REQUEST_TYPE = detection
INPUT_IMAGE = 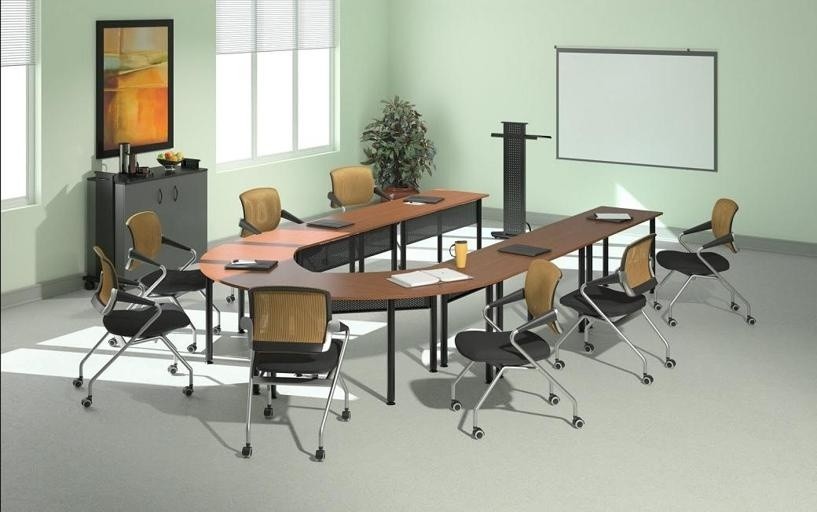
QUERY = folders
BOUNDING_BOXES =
[224,257,278,271]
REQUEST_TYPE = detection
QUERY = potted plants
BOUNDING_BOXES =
[362,94,439,203]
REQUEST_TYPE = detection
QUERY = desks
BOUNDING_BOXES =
[199,189,662,405]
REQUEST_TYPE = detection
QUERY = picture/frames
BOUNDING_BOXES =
[95,18,173,159]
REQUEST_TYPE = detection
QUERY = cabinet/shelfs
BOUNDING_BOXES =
[85,160,207,291]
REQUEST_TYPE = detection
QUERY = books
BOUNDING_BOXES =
[224,259,279,272]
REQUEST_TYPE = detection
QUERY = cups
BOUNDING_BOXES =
[449,241,468,269]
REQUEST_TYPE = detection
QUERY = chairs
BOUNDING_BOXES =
[125,211,222,354]
[450,260,584,438]
[560,235,676,385]
[226,187,305,303]
[242,286,353,462]
[74,245,194,408]
[653,199,756,328]
[326,167,391,209]
